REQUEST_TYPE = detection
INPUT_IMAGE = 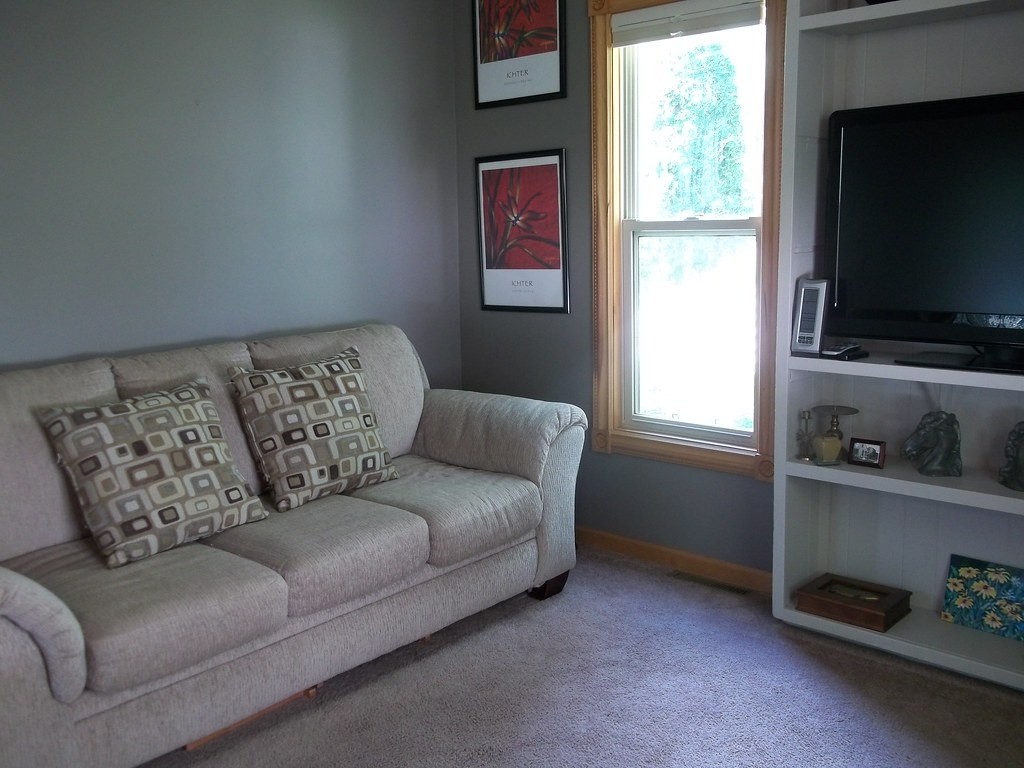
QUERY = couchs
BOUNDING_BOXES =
[0,323,588,768]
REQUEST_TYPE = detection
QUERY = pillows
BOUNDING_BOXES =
[35,375,269,569]
[229,345,399,512]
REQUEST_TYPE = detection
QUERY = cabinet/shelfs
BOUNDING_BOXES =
[771,0,1024,692]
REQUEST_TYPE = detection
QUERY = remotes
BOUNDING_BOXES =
[822,342,862,356]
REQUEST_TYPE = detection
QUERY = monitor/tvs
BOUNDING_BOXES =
[825,92,1024,374]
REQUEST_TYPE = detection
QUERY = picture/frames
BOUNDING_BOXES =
[847,438,886,469]
[475,148,570,314]
[471,0,566,110]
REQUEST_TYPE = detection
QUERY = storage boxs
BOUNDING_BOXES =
[795,572,912,633]
[941,554,1024,642]
